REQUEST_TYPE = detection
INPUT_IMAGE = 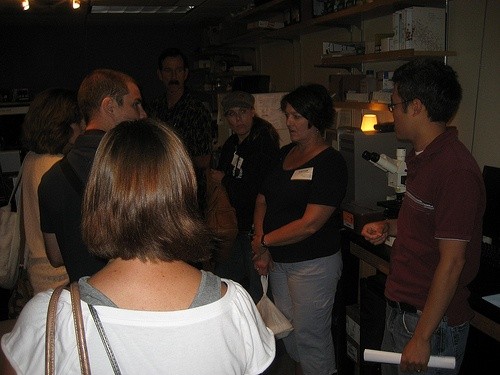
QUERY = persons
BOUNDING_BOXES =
[37,69,147,284]
[146,49,211,209]
[0,116,277,375]
[212,91,279,206]
[13,89,84,296]
[359,59,486,375]
[250,83,348,375]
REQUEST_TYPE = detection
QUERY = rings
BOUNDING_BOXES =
[417,368,421,374]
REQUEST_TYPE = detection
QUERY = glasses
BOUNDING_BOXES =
[387,99,412,112]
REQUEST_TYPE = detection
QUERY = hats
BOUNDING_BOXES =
[221,92,255,111]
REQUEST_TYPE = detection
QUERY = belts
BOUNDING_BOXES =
[385,298,418,315]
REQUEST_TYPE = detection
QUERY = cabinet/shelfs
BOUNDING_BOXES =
[201,0,464,73]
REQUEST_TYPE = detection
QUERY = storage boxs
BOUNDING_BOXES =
[191,51,254,73]
[329,75,401,135]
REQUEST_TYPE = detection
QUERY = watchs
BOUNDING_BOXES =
[260,233,271,250]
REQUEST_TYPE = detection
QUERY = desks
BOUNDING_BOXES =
[340,228,500,375]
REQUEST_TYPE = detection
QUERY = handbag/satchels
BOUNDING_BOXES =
[0,150,35,318]
